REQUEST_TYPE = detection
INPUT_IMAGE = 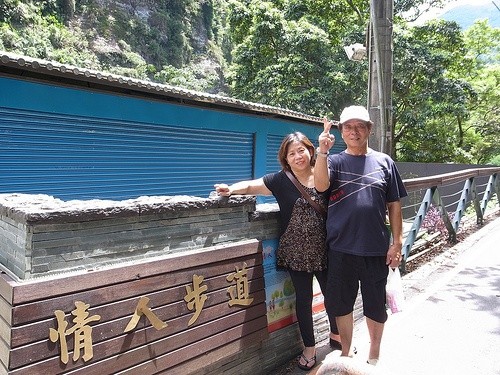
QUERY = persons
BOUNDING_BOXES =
[214,132,358,371]
[314,105,408,368]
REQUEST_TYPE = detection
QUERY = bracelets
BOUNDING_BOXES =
[317,147,329,157]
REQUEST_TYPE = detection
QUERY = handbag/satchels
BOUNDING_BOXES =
[385,264,403,314]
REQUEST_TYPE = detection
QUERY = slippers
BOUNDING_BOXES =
[297,351,316,371]
[330,338,357,354]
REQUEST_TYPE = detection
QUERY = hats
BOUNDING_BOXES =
[339,104,374,125]
